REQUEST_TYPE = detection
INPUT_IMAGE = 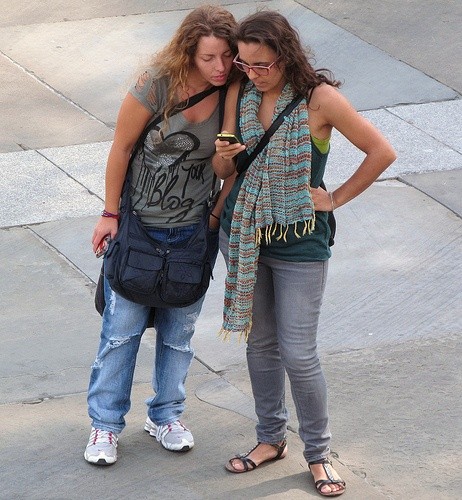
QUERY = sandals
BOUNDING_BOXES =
[225,439,288,473]
[308,459,346,496]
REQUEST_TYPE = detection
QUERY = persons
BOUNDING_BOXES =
[81,5,243,466]
[210,10,398,499]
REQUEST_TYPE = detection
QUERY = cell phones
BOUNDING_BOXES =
[217,134,241,145]
[95,234,111,258]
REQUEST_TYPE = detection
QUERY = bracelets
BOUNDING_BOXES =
[102,210,119,219]
[329,191,335,211]
[210,213,220,221]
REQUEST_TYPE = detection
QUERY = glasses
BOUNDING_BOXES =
[233,53,280,75]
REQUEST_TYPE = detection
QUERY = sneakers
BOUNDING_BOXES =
[143,417,195,452]
[84,427,118,465]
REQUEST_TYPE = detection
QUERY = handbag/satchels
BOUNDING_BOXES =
[104,209,213,308]
[95,261,157,329]
